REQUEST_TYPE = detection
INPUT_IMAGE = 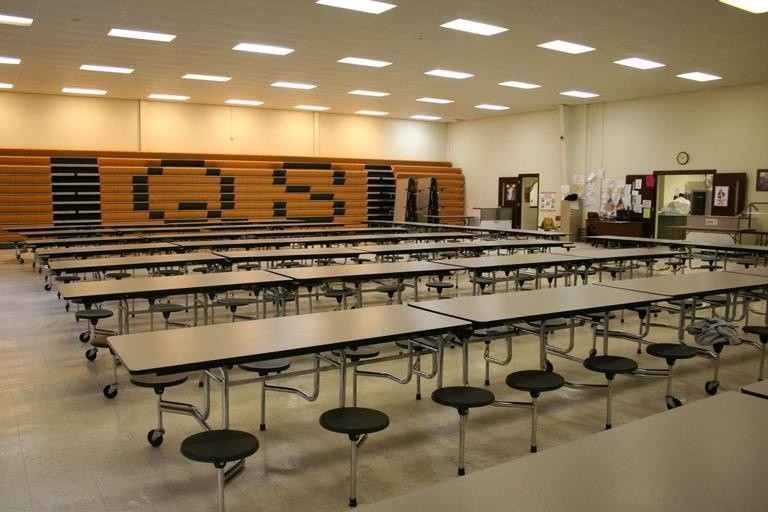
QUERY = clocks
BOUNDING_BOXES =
[677,152,689,165]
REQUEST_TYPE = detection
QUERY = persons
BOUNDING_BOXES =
[665,196,677,212]
[672,193,691,216]
[717,188,727,205]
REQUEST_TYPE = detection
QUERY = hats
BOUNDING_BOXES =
[674,186,684,197]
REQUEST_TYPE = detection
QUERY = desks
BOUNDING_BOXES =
[334,389,766,512]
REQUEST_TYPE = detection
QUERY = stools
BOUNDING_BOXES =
[9,211,766,450]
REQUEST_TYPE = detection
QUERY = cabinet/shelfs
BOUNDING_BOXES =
[559,200,583,244]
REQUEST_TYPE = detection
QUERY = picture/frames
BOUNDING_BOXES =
[756,168,768,191]
